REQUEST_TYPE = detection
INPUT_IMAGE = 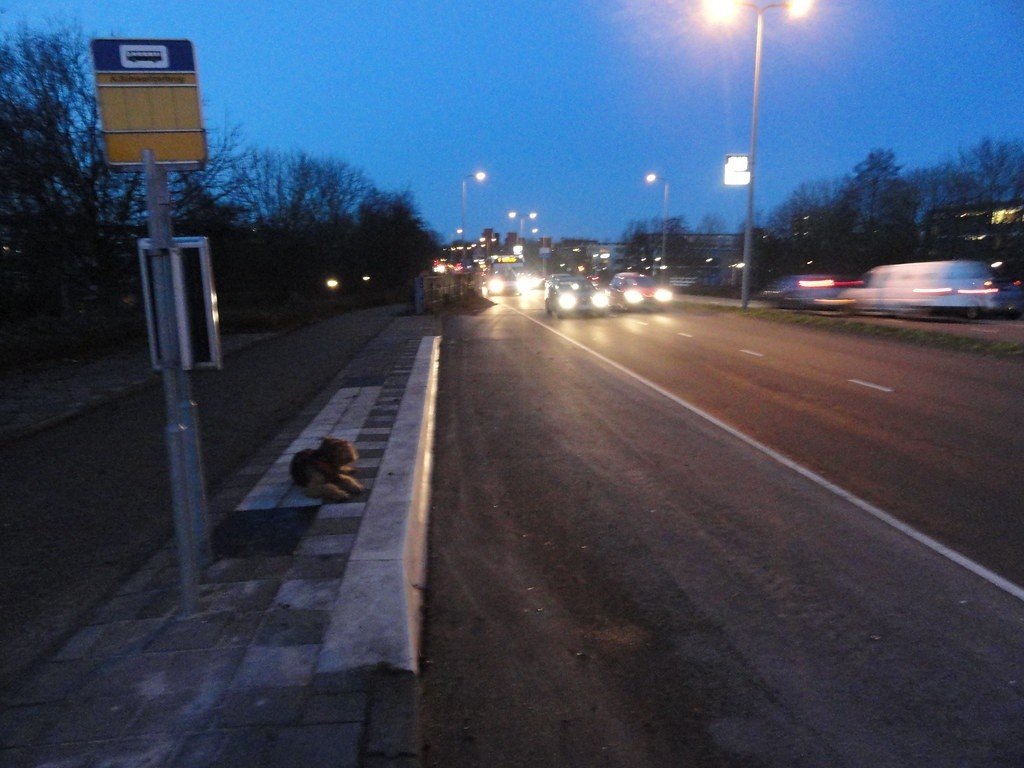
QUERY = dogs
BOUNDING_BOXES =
[288,435,365,501]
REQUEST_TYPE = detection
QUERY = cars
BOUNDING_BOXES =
[482,261,614,319]
[781,258,1024,320]
[614,271,677,316]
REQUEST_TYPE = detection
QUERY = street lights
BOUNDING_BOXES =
[695,0,818,310]
[646,173,669,263]
[509,210,538,256]
[459,168,486,261]
